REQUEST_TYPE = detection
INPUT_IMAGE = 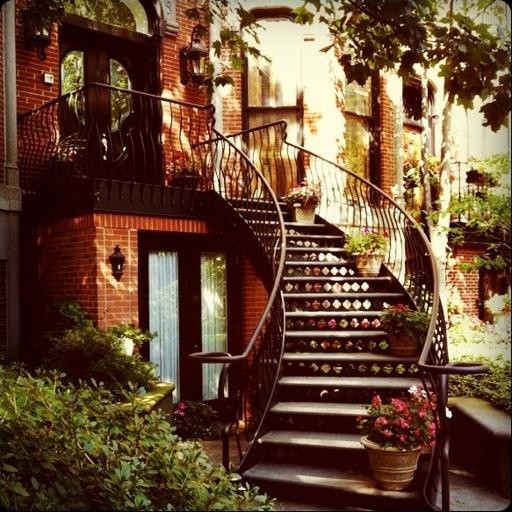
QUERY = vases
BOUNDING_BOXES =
[360,433,424,490]
[351,251,385,277]
[388,329,424,357]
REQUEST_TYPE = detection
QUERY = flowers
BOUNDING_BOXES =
[343,228,390,260]
[379,304,432,343]
[356,386,453,452]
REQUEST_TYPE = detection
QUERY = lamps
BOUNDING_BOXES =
[180,25,209,87]
[108,245,126,281]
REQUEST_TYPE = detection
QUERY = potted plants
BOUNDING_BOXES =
[280,185,324,223]
